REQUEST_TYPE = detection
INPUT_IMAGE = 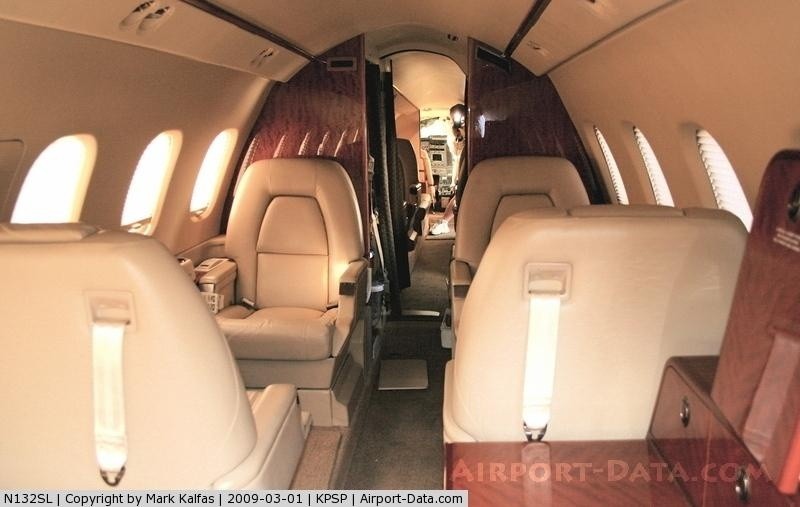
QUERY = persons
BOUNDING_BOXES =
[429,123,466,235]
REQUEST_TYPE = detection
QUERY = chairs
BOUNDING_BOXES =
[437,155,750,449]
[0,138,429,490]
